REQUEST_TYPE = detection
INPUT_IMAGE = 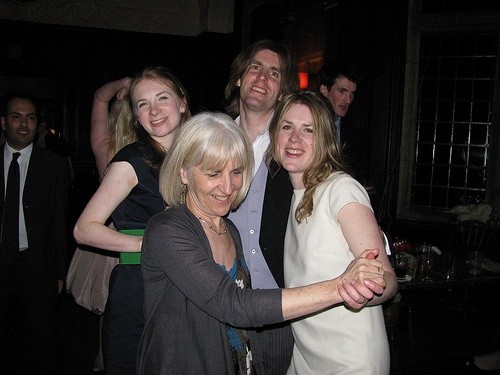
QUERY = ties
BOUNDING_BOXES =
[1,152,22,262]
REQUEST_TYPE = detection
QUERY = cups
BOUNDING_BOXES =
[469,251,484,275]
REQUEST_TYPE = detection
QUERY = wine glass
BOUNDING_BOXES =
[417,243,434,282]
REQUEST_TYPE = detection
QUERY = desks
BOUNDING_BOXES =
[395,265,500,351]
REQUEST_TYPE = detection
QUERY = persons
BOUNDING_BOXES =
[71,64,194,375]
[307,59,362,176]
[0,89,72,375]
[63,75,138,375]
[135,111,386,375]
[34,110,51,150]
[260,88,399,375]
[222,39,303,375]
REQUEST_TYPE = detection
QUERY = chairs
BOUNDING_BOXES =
[431,221,493,352]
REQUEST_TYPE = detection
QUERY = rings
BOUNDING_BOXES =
[360,298,366,304]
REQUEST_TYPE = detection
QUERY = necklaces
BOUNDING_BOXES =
[195,213,227,235]
